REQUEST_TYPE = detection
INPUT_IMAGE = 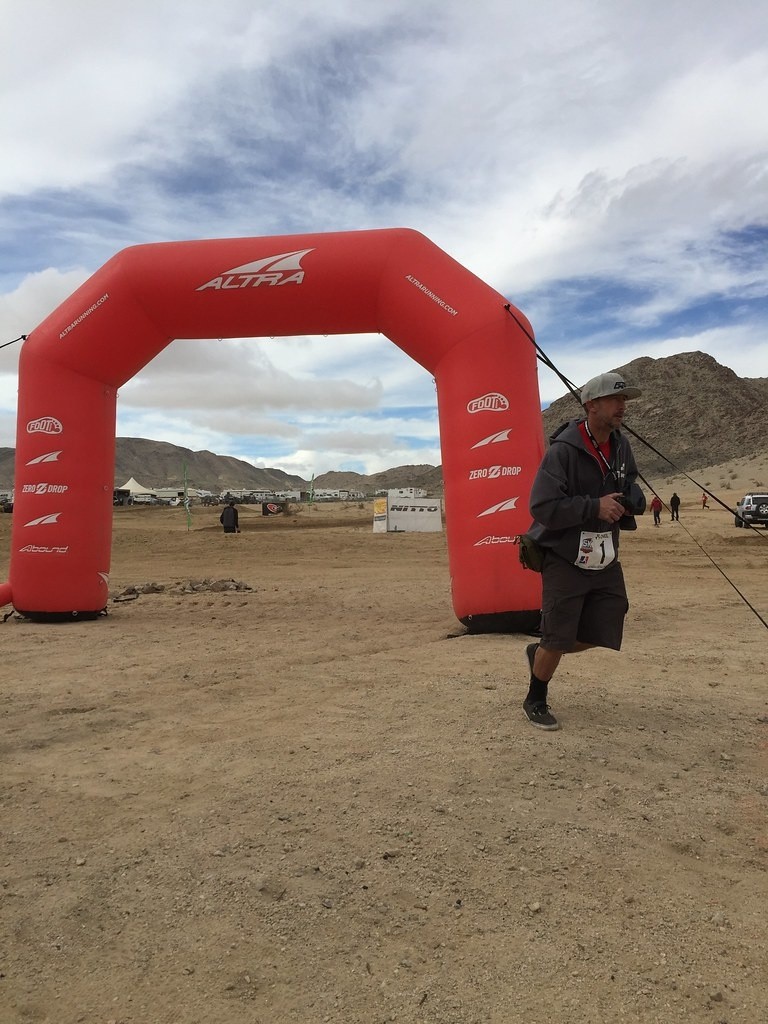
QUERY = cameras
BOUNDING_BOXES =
[614,484,647,516]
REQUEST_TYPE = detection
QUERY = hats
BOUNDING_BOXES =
[581,373,642,406]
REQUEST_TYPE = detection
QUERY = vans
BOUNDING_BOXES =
[275,494,291,502]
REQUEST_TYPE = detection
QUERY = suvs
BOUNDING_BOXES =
[734,491,768,528]
[133,495,152,505]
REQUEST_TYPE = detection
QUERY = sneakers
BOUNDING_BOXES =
[525,643,540,687]
[523,694,558,731]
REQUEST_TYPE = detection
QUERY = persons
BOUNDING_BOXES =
[650,496,662,525]
[219,500,239,533]
[702,493,709,509]
[670,493,680,521]
[521,373,643,731]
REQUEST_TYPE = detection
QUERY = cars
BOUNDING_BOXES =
[203,496,220,507]
[113,496,120,506]
[241,498,262,504]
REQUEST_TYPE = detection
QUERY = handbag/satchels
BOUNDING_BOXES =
[513,534,543,572]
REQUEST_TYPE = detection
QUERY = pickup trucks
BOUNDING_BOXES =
[169,496,194,507]
